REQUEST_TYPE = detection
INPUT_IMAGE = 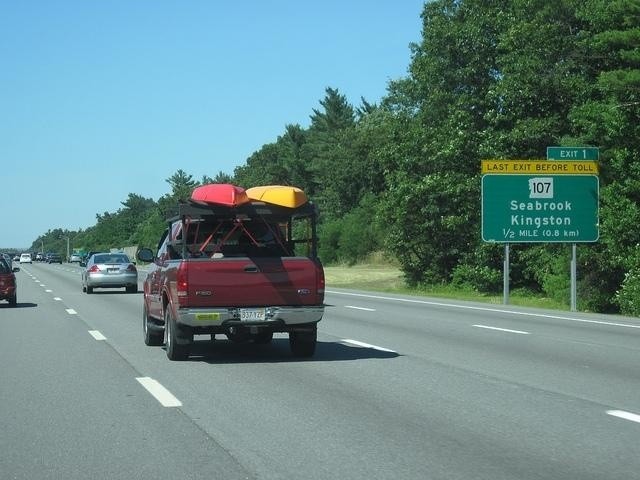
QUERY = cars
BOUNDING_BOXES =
[79,252,137,294]
[36,252,62,264]
[70,252,101,266]
[0,252,33,306]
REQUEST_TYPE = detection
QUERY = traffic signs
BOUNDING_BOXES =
[482,174,600,243]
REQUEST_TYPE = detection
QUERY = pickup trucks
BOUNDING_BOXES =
[137,212,326,361]
